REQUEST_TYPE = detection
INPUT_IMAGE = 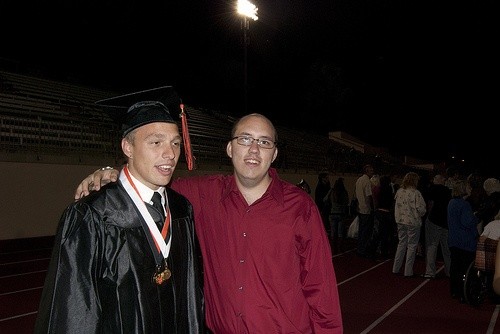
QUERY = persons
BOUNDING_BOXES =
[34,85,206,334]
[315,164,500,308]
[75,114,344,334]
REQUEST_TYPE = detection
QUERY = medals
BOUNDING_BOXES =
[162,268,171,281]
[151,271,164,286]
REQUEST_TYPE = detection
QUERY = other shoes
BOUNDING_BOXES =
[404,272,417,278]
[392,273,401,275]
[420,272,435,280]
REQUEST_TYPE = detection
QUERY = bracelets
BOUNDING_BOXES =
[95,166,115,171]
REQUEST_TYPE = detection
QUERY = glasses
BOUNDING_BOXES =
[230,135,277,149]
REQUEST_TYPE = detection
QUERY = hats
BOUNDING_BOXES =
[95,86,196,171]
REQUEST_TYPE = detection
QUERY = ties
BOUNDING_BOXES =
[151,192,165,225]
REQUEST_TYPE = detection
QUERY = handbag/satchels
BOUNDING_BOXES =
[347,215,359,239]
